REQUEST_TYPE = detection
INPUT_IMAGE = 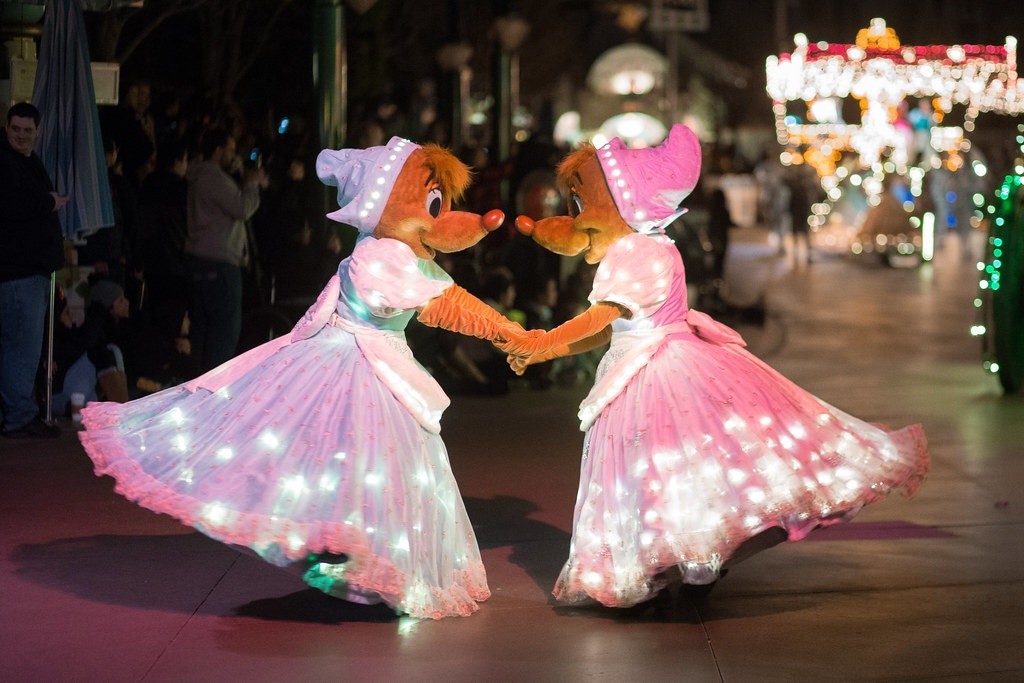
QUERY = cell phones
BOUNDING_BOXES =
[250,148,263,169]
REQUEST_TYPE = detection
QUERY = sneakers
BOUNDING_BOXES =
[3,413,60,440]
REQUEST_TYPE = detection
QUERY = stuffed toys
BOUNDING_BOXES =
[77,136,547,619]
[492,122,930,608]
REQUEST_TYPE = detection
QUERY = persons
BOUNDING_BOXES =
[0,100,743,436]
[755,144,988,268]
